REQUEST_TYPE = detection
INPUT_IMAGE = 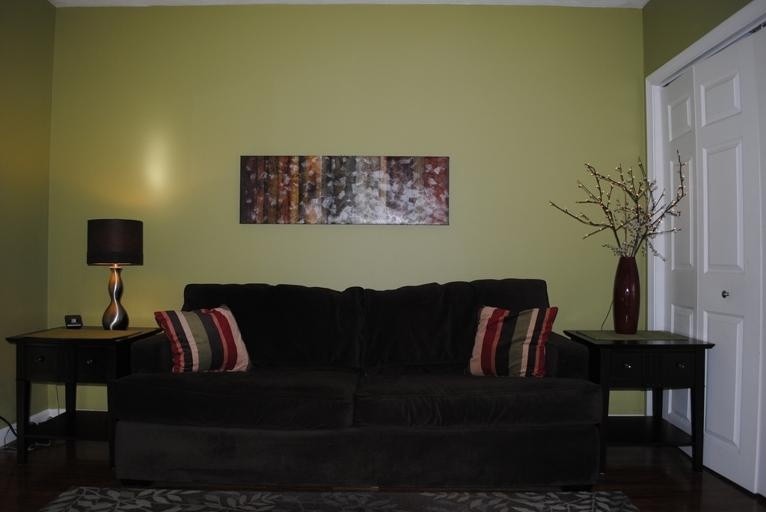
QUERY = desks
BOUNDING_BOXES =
[563,329,716,490]
[5,324,167,465]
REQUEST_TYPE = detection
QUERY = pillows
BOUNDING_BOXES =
[154,304,253,375]
[464,304,557,380]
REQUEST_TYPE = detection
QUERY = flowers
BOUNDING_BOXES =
[550,148,705,263]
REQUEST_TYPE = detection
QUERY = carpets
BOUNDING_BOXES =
[41,488,642,512]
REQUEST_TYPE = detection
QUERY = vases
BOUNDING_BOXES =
[609,256,646,333]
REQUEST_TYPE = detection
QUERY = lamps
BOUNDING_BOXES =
[86,218,143,330]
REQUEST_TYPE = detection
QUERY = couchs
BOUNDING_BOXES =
[111,278,606,484]
